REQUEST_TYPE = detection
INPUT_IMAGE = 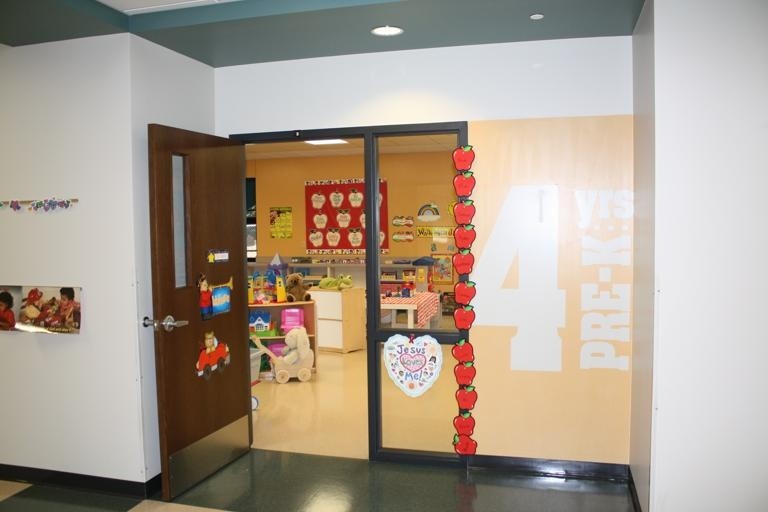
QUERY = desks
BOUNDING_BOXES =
[382,289,433,331]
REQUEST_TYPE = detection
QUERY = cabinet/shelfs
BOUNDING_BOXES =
[307,285,368,355]
[246,298,319,376]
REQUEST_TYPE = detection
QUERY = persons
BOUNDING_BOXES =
[0,288,74,331]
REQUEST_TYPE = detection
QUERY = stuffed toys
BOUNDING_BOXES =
[285,274,313,302]
